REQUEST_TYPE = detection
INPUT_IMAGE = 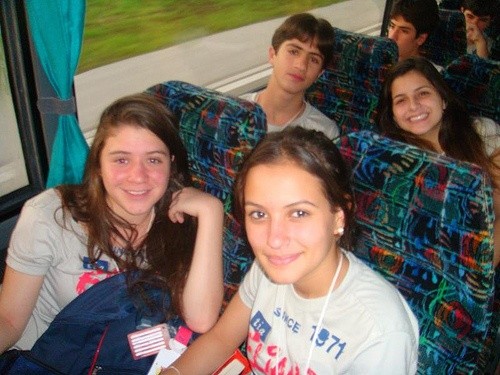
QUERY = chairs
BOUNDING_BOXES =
[387,9,466,68]
[144,80,267,319]
[439,1,500,46]
[304,26,399,137]
[328,129,500,375]
[440,53,500,125]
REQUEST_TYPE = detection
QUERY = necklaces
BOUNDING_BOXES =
[255,88,305,133]
[275,251,344,375]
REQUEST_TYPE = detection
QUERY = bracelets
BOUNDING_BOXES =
[159,365,181,375]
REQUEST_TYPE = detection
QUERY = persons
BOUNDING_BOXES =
[238,14,340,141]
[387,0,444,72]
[379,57,500,268]
[158,124,420,375]
[0,95,223,375]
[460,0,500,61]
